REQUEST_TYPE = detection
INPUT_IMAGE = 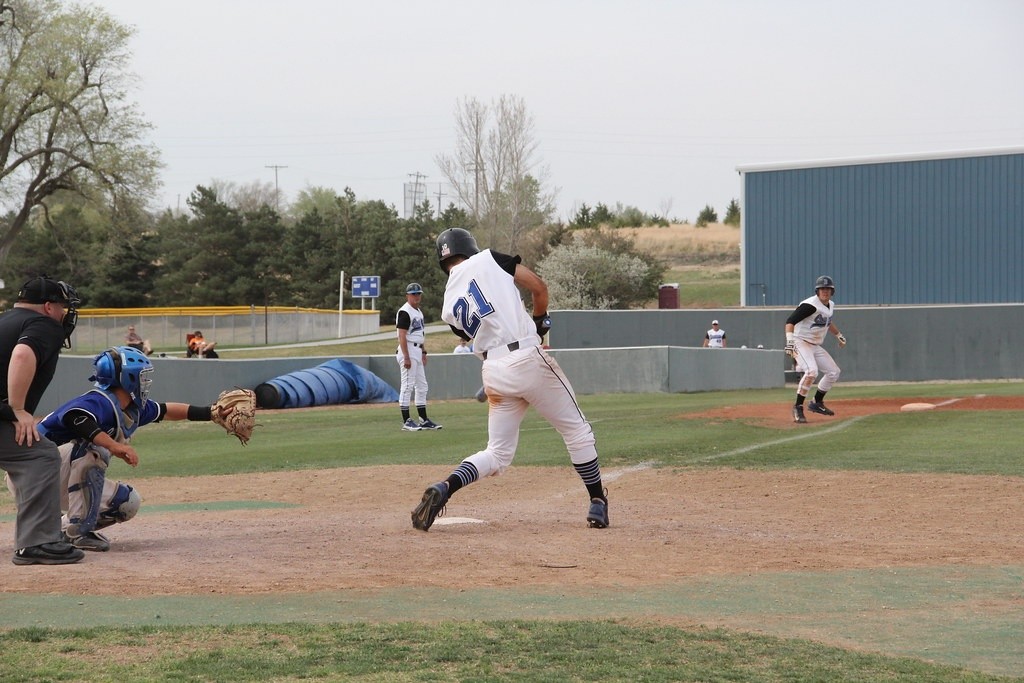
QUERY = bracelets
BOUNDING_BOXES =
[422,351,427,354]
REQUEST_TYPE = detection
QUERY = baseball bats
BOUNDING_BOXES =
[474,319,552,403]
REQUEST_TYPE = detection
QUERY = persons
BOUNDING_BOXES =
[10,350,256,552]
[784,276,846,423]
[124,325,154,355]
[0,277,85,567]
[453,338,470,352]
[189,331,216,358]
[703,320,727,347]
[411,228,609,531]
[395,283,443,432]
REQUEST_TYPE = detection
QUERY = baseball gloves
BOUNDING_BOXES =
[209,388,257,443]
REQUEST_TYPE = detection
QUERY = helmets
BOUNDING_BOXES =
[89,346,154,411]
[815,276,835,295]
[436,228,479,275]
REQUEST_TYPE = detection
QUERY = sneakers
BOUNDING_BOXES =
[793,405,806,423]
[418,420,442,429]
[12,542,85,564]
[401,418,422,431]
[61,531,109,551]
[587,488,609,528]
[411,481,448,531]
[807,401,834,416]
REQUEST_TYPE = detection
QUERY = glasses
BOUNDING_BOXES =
[412,293,421,296]
[713,324,718,325]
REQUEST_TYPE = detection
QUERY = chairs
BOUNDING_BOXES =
[186,334,199,358]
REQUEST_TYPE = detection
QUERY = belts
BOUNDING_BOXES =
[483,342,519,359]
[415,343,423,348]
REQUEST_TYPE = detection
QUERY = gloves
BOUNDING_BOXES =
[785,340,798,358]
[533,311,550,335]
[835,332,846,348]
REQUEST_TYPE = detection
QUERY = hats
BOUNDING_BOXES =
[712,320,718,324]
[406,283,423,294]
[15,279,67,304]
